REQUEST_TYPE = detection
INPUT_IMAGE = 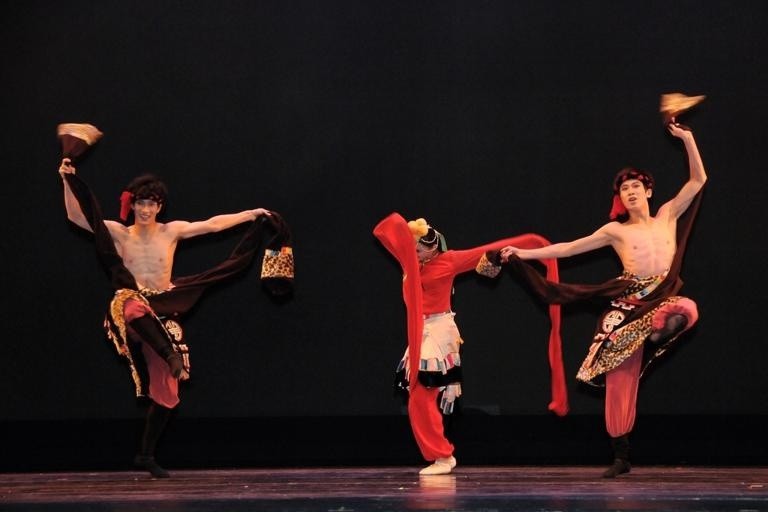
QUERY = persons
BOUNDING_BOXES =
[57,156,272,479]
[371,212,549,475]
[500,115,708,479]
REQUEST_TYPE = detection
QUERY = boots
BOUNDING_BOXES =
[649,307,687,342]
[143,393,179,478]
[128,312,182,378]
[598,431,635,479]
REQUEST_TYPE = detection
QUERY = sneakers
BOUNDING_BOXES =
[419,455,457,476]
[445,384,464,404]
